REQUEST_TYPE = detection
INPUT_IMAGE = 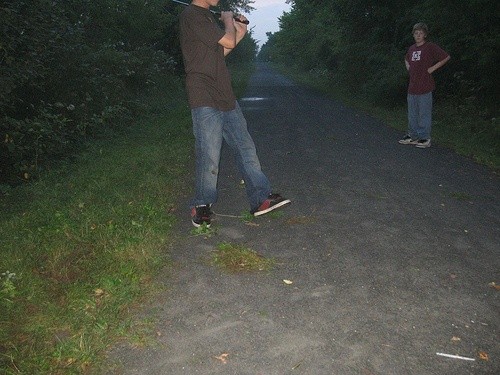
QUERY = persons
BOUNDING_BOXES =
[399,22,451,148]
[178,0,291,228]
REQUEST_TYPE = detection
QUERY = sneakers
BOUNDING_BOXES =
[253,193,291,217]
[191,204,212,229]
[415,139,431,149]
[398,136,419,145]
